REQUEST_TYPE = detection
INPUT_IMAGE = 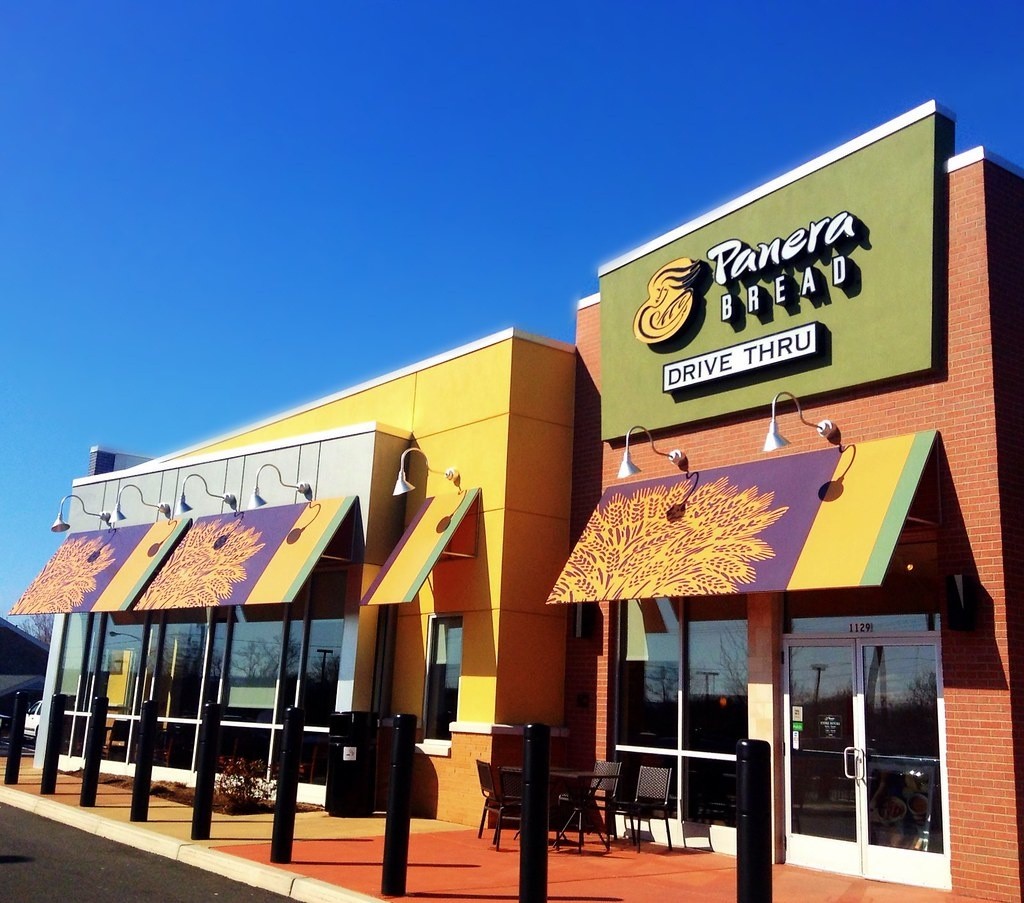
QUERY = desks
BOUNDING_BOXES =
[514,766,620,852]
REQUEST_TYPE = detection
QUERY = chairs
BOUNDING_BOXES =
[476,759,512,845]
[608,765,674,854]
[555,761,623,844]
[496,765,523,851]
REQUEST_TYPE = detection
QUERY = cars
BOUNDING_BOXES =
[23,699,42,743]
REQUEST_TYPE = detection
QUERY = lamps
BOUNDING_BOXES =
[249,464,310,510]
[174,474,236,518]
[107,484,170,523]
[616,425,687,480]
[393,446,459,496]
[51,494,110,532]
[763,391,837,453]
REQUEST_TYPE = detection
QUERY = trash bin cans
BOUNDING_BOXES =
[329,711,379,817]
